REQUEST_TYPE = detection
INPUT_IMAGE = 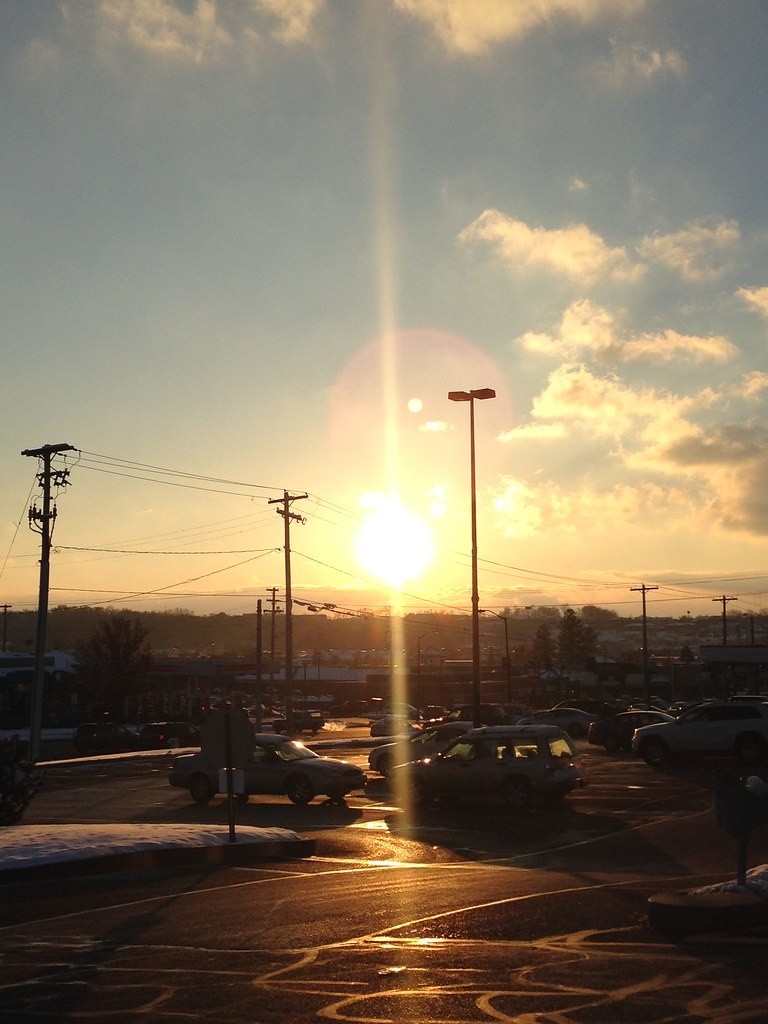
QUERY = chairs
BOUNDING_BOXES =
[501,749,506,757]
[527,748,538,756]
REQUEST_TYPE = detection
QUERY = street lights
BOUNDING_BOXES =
[416,629,438,722]
[478,609,510,725]
[449,389,496,728]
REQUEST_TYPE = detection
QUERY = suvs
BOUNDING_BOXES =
[385,724,582,810]
[631,701,768,766]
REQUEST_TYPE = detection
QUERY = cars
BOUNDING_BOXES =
[139,723,204,751]
[168,736,368,805]
[71,722,141,754]
[212,691,768,748]
